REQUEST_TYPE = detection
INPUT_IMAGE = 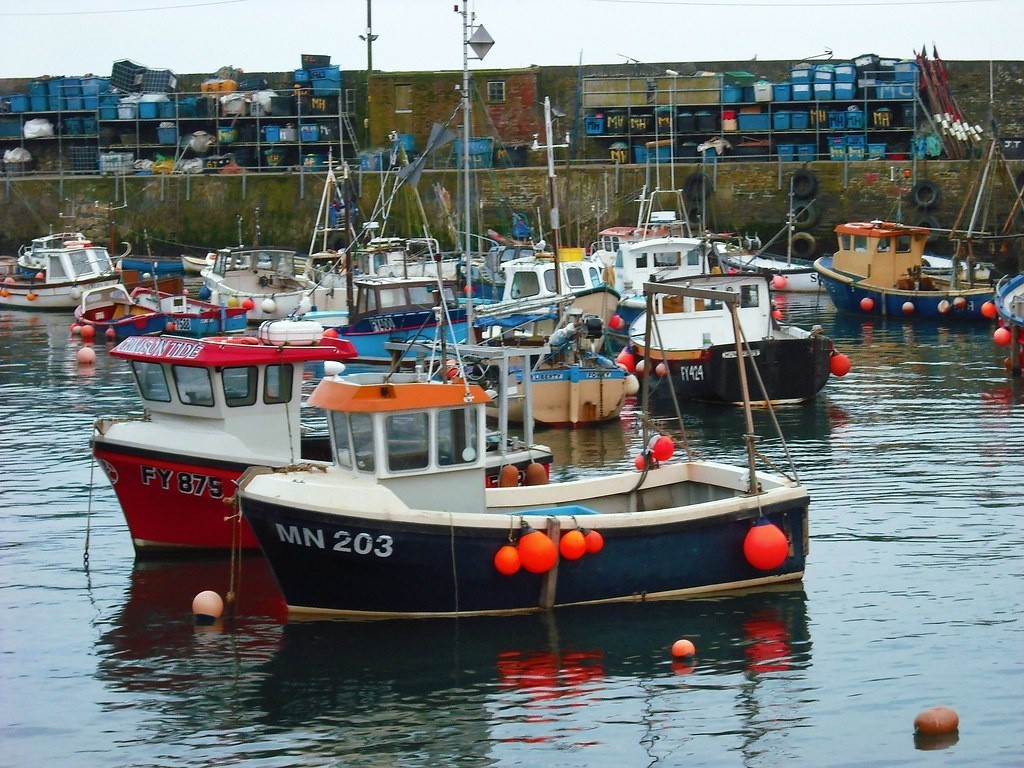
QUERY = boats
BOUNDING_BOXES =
[180,85,712,431]
[994,274,1024,372]
[230,223,810,620]
[90,0,554,562]
[0,224,121,312]
[920,255,996,283]
[130,286,247,339]
[814,118,1024,322]
[628,147,834,407]
[113,258,184,273]
[75,283,164,340]
[713,177,828,293]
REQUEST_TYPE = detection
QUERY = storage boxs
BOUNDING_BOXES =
[0,54,353,176]
[608,148,629,165]
[605,110,628,133]
[629,115,654,134]
[701,147,719,163]
[677,115,696,133]
[453,138,529,170]
[676,146,701,163]
[657,110,675,133]
[633,145,670,164]
[695,113,717,132]
[999,136,1024,160]
[359,133,421,171]
[721,54,921,162]
[583,114,605,135]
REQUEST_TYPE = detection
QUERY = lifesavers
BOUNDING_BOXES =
[200,337,258,345]
[327,232,356,253]
[792,201,817,229]
[913,216,940,244]
[62,240,92,248]
[790,232,816,259]
[683,173,711,201]
[911,181,941,210]
[1016,170,1024,231]
[790,170,816,199]
[684,202,710,230]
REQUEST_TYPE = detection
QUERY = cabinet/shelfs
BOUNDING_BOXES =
[0,87,356,177]
[582,70,924,164]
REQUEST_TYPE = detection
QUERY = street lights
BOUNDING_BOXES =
[359,0,379,74]
